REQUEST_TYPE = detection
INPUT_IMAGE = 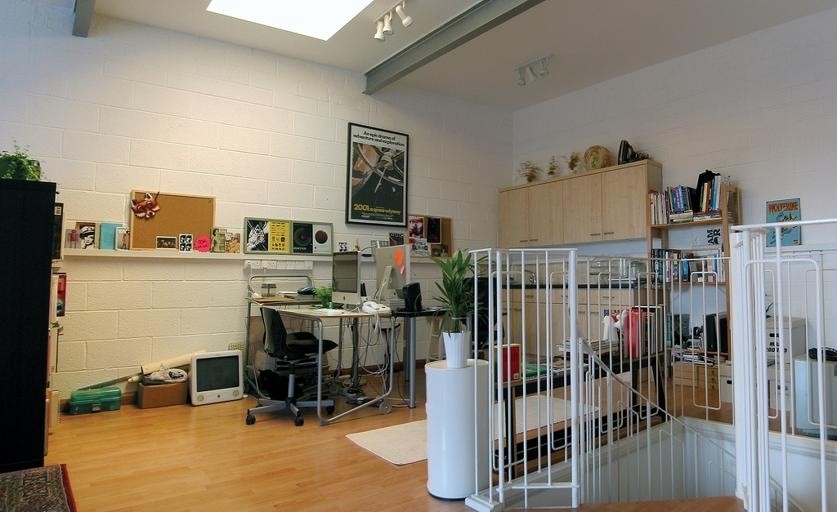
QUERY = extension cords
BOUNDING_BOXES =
[349,388,357,397]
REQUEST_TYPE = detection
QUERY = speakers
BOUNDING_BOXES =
[401,282,422,311]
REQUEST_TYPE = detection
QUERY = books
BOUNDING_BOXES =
[646,174,735,285]
[492,302,731,387]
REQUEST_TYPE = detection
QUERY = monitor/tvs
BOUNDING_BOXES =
[332,251,361,310]
[375,245,410,299]
[188,350,243,406]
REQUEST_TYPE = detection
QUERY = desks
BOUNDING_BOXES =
[424,358,491,500]
[390,306,452,409]
[263,307,394,426]
[493,354,670,482]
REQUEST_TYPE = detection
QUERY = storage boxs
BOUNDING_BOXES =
[765,316,806,360]
[68,387,120,415]
[718,363,732,403]
[767,363,792,411]
[136,381,188,409]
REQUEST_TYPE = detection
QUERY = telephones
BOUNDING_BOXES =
[362,301,391,314]
[809,347,837,361]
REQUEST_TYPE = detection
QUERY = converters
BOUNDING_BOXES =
[346,389,358,394]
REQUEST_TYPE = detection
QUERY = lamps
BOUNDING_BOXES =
[513,51,552,87]
[372,1,414,40]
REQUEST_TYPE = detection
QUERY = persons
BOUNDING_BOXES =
[79,225,95,249]
[210,229,222,251]
[179,235,191,251]
[412,218,424,237]
[440,247,448,256]
[409,219,417,237]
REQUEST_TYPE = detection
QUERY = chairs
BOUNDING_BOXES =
[244,306,336,426]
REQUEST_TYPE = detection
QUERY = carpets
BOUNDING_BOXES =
[0,464,77,511]
[344,393,602,468]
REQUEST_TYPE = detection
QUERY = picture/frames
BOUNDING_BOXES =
[129,189,217,253]
[243,215,334,256]
[344,121,409,228]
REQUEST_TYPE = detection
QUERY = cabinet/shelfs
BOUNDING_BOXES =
[497,174,563,247]
[672,363,718,392]
[513,287,546,356]
[563,158,662,246]
[589,287,670,339]
[551,286,586,357]
[0,178,58,473]
[644,184,742,360]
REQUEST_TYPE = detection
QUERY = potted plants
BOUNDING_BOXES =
[424,246,497,369]
[516,156,541,183]
[560,149,583,174]
[545,156,560,177]
[311,282,337,309]
[0,139,40,180]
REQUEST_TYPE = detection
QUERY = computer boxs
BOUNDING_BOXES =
[371,298,406,311]
